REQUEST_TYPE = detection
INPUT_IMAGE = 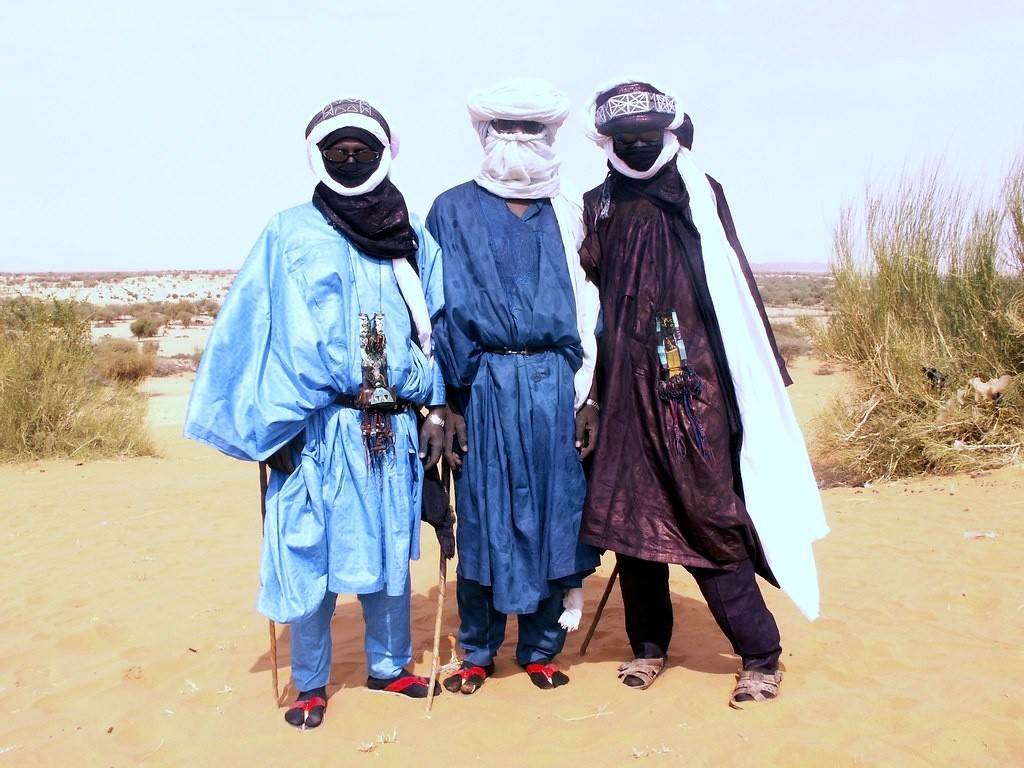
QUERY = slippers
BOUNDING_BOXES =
[620,657,663,690]
[284,697,326,731]
[730,661,786,710]
[443,662,495,694]
[369,675,438,698]
[521,658,569,690]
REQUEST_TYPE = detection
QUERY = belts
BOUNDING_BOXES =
[482,345,549,356]
[332,393,411,414]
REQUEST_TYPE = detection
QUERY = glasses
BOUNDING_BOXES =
[323,148,379,163]
[495,120,540,134]
[614,129,661,144]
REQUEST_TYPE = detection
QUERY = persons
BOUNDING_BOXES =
[182,72,831,731]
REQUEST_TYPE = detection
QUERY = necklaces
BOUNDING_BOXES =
[632,202,718,466]
[347,243,395,469]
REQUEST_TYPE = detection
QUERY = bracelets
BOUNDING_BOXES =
[587,399,601,410]
[428,414,446,428]
